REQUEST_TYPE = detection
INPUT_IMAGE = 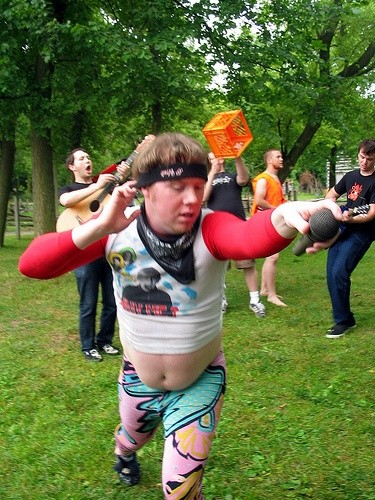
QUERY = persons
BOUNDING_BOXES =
[112,251,174,317]
[324,139,375,339]
[18,133,343,500]
[58,148,132,362]
[250,150,288,307]
[201,143,267,320]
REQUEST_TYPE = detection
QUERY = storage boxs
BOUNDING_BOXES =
[201,110,253,159]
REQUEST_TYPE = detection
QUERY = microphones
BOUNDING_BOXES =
[294,208,340,256]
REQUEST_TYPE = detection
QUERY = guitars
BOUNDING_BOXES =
[341,204,370,217]
[55,134,157,235]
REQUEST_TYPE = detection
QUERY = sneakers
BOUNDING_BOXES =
[83,348,103,362]
[102,344,118,355]
[326,320,357,338]
[114,454,141,486]
[250,299,266,318]
[221,296,228,314]
